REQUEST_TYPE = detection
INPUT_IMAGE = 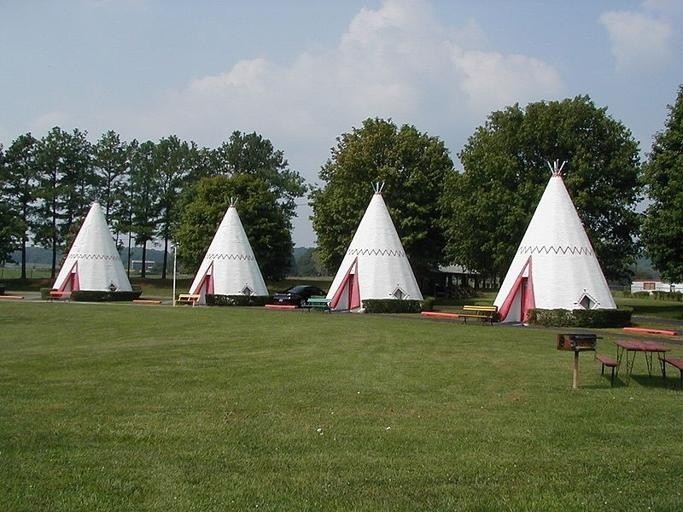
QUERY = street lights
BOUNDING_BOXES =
[170,245,176,307]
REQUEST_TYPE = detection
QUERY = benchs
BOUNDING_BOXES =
[591,337,683,385]
[304,297,333,313]
[458,305,498,326]
[45,290,73,304]
[175,293,201,306]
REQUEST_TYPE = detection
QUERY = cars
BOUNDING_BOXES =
[271,284,327,308]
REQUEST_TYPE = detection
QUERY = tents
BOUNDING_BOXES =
[45,200,136,299]
[490,169,617,326]
[324,192,424,312]
[182,203,268,304]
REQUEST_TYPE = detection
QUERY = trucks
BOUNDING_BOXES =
[132,260,155,273]
